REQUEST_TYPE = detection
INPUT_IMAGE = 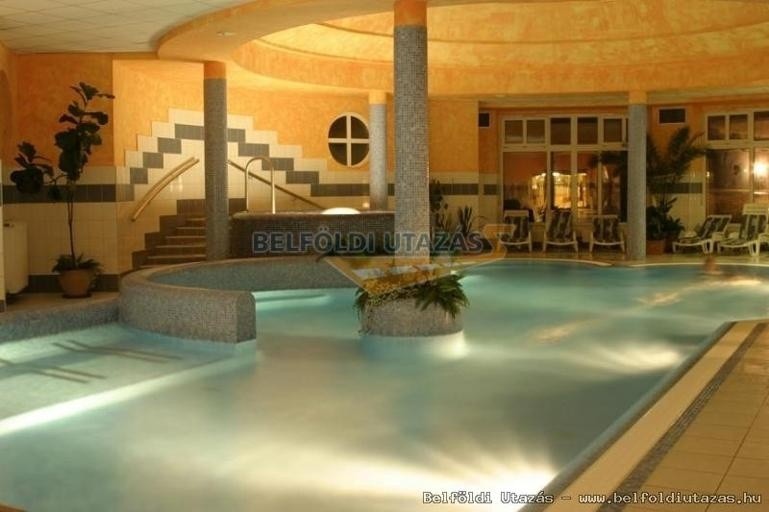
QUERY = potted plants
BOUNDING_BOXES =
[588,124,710,256]
[10,82,115,299]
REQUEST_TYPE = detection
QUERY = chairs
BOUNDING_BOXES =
[671,214,733,255]
[496,209,533,254]
[717,204,769,257]
[542,209,579,253]
